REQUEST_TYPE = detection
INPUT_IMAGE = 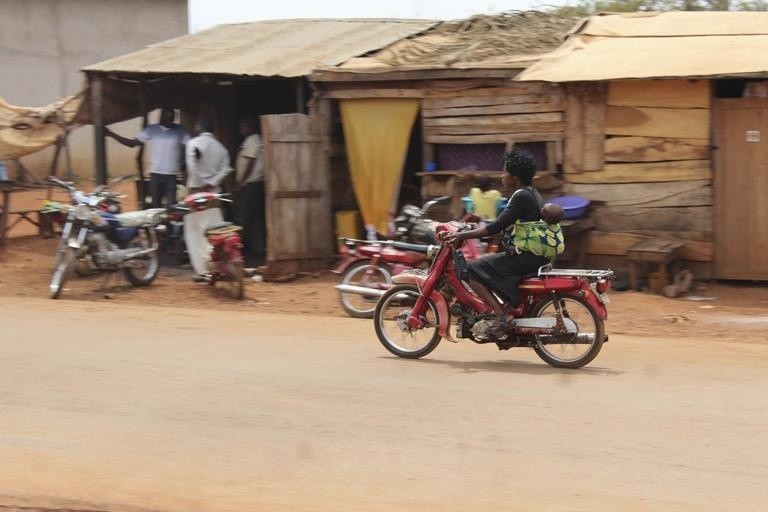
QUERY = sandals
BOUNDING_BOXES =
[484,317,517,334]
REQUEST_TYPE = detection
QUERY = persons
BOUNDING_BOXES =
[443,146,551,333]
[183,113,232,284]
[515,202,565,259]
[103,106,188,209]
[234,114,264,271]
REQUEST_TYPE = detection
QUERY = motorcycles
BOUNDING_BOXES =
[43,173,244,298]
[384,194,502,253]
[373,204,615,370]
[327,214,503,319]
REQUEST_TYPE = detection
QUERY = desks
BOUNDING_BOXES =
[7,208,40,231]
[558,212,597,238]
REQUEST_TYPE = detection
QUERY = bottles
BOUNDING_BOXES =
[457,251,466,279]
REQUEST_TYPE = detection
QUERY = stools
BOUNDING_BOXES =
[39,208,60,238]
[625,238,686,292]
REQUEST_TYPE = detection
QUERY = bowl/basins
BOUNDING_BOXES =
[462,196,507,214]
[548,196,589,219]
